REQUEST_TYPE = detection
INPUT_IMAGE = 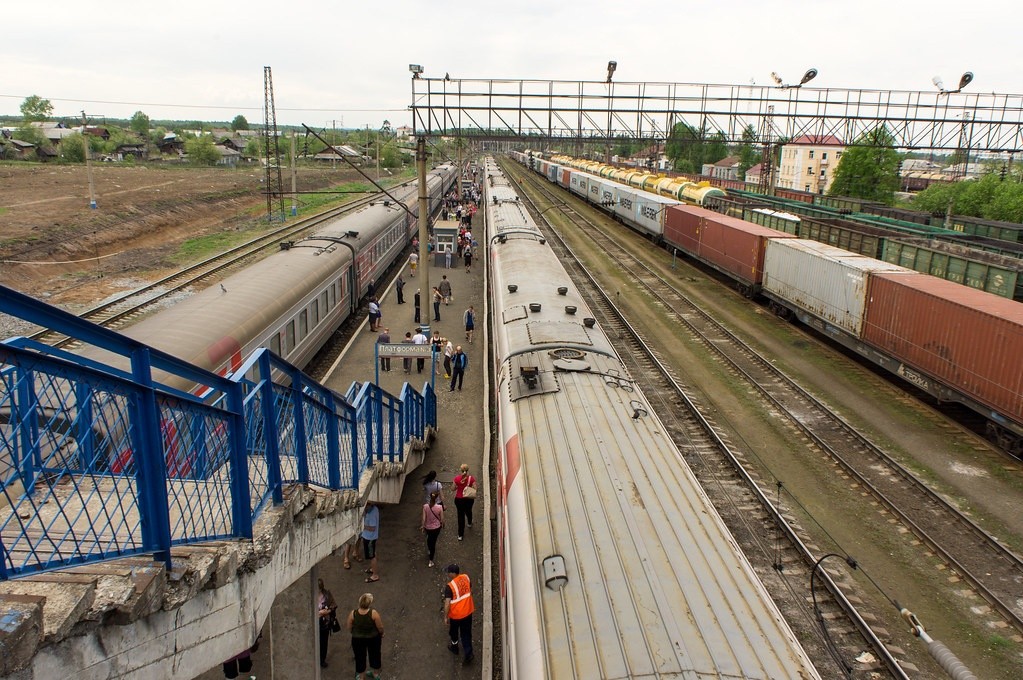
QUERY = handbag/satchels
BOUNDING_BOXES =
[462,476,477,499]
[331,618,341,633]
[449,295,454,301]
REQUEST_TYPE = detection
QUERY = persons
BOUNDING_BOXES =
[396,160,483,323]
[463,305,475,345]
[444,564,476,654]
[412,328,428,374]
[368,279,377,302]
[422,490,445,567]
[448,345,468,392]
[451,463,478,541]
[423,470,447,511]
[347,592,386,680]
[223,629,262,680]
[400,331,415,375]
[318,578,341,668]
[430,331,444,375]
[361,502,380,583]
[369,295,383,332]
[376,328,391,372]
[443,337,454,379]
[344,533,363,569]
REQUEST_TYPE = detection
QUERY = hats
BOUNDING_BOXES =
[444,564,460,575]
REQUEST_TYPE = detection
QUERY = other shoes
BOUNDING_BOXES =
[447,644,459,656]
[428,560,434,568]
[355,671,360,680]
[366,670,380,680]
[436,370,441,375]
[462,653,475,666]
[466,337,472,345]
[399,301,406,303]
[443,373,451,379]
[433,319,438,322]
[466,270,470,273]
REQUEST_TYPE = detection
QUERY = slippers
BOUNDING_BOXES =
[352,554,364,563]
[364,568,373,573]
[343,558,351,569]
[364,575,378,583]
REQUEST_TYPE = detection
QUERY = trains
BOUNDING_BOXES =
[482,148,1023,680]
[0,154,469,491]
